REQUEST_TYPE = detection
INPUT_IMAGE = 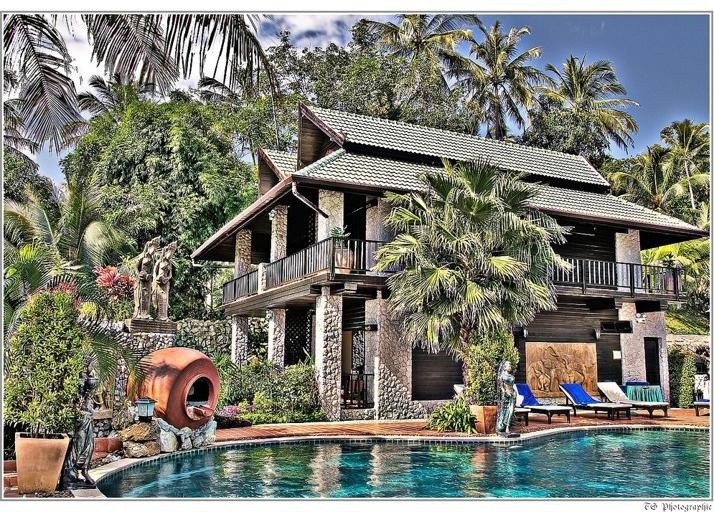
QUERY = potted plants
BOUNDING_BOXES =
[2,288,90,497]
[330,224,355,275]
[461,332,521,436]
[662,252,679,294]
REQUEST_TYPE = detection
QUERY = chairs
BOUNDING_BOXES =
[453,381,712,427]
[342,379,364,409]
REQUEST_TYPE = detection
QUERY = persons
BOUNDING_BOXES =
[131,238,176,320]
[495,361,517,432]
[65,361,100,486]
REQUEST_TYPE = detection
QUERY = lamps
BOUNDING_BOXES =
[135,396,158,423]
